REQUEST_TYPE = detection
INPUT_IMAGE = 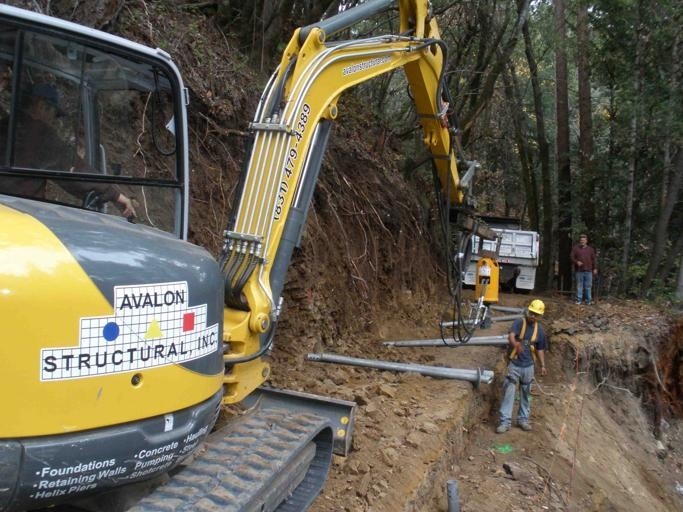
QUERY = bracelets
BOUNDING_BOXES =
[540,364,546,368]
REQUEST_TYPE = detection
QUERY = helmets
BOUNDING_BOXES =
[528,299,546,316]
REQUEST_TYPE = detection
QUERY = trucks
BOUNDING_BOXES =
[453,211,543,294]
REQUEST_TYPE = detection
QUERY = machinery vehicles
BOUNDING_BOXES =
[0,1,507,511]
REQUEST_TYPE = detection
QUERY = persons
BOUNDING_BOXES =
[495,300,547,433]
[0,81,138,218]
[570,235,598,306]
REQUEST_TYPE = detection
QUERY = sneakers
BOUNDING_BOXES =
[517,420,532,431]
[496,422,512,434]
[575,301,591,306]
